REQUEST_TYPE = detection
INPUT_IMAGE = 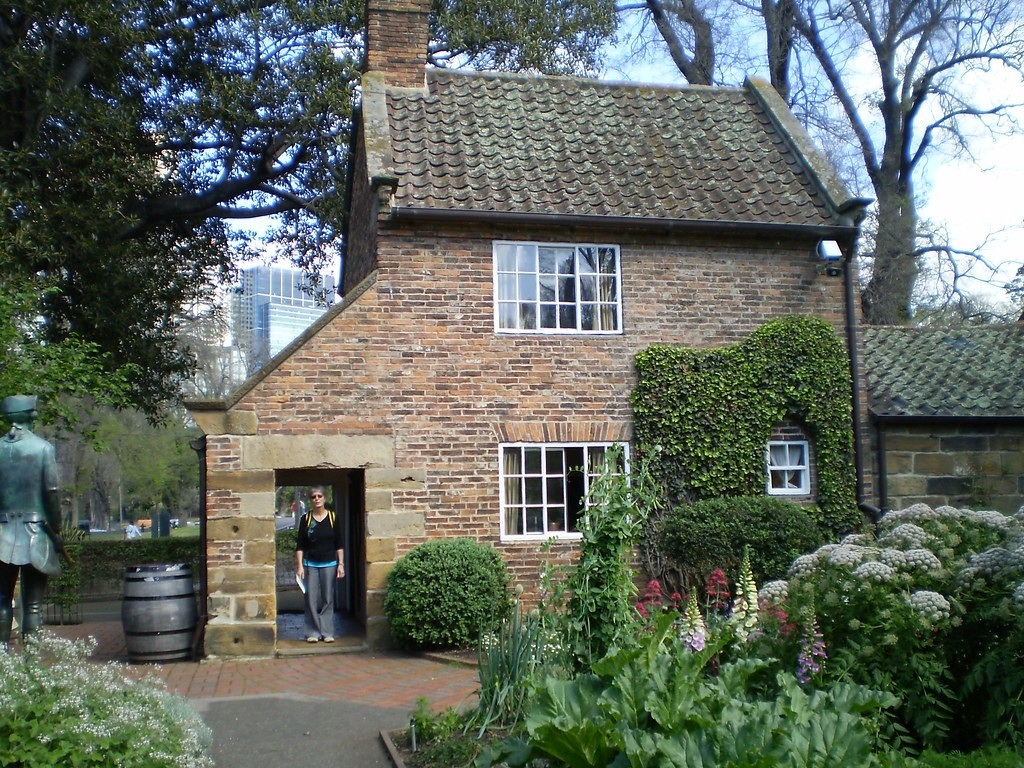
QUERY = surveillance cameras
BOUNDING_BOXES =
[819,240,842,260]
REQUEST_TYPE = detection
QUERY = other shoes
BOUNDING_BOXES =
[324,637,334,643]
[306,637,318,643]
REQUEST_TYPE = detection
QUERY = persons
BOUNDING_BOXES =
[124,521,143,540]
[294,484,346,643]
[0,395,65,646]
[299,499,305,515]
[291,500,297,522]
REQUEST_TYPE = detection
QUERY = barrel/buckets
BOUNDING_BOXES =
[119,564,197,665]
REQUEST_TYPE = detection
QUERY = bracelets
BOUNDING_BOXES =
[338,563,344,566]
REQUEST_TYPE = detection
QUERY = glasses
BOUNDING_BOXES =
[311,495,323,499]
